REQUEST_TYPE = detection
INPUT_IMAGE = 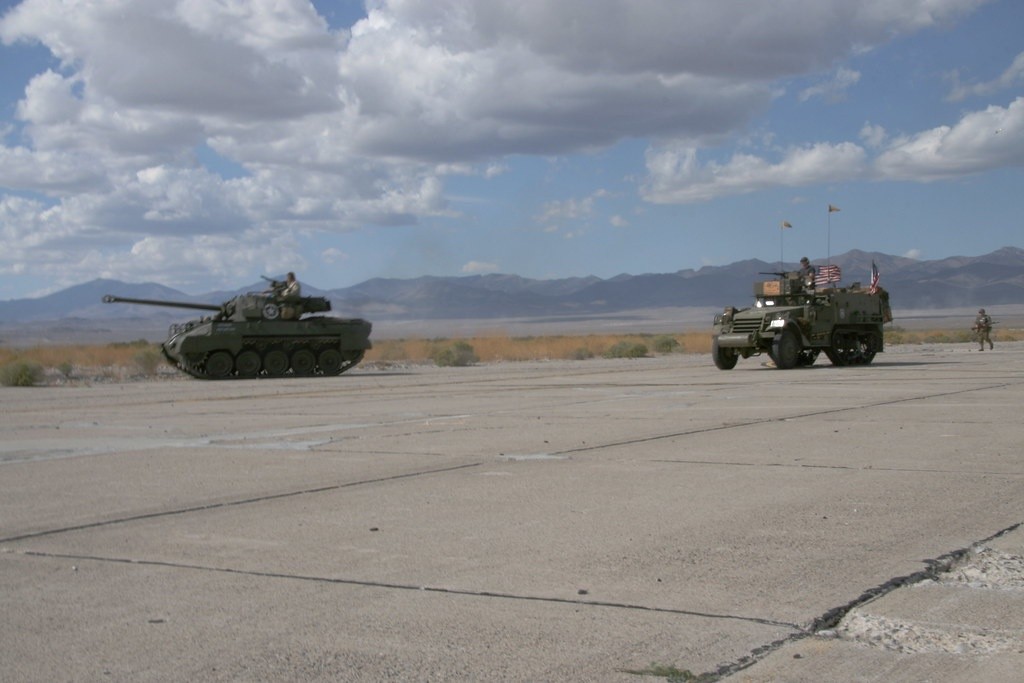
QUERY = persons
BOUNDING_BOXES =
[796,256,816,290]
[281,272,300,317]
[975,309,993,351]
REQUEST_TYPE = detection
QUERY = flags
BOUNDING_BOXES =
[829,204,840,212]
[784,221,792,228]
[811,264,840,284]
[870,261,880,294]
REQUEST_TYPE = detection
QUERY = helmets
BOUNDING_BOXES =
[979,309,986,313]
[800,257,809,264]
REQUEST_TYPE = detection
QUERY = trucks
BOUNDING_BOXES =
[710,271,893,371]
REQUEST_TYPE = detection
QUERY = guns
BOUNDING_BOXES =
[261,275,288,288]
[971,321,1000,331]
[757,271,790,280]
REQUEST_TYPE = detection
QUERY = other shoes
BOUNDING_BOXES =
[990,345,994,350]
[979,348,984,351]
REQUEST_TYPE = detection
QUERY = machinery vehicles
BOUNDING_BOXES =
[100,281,373,380]
